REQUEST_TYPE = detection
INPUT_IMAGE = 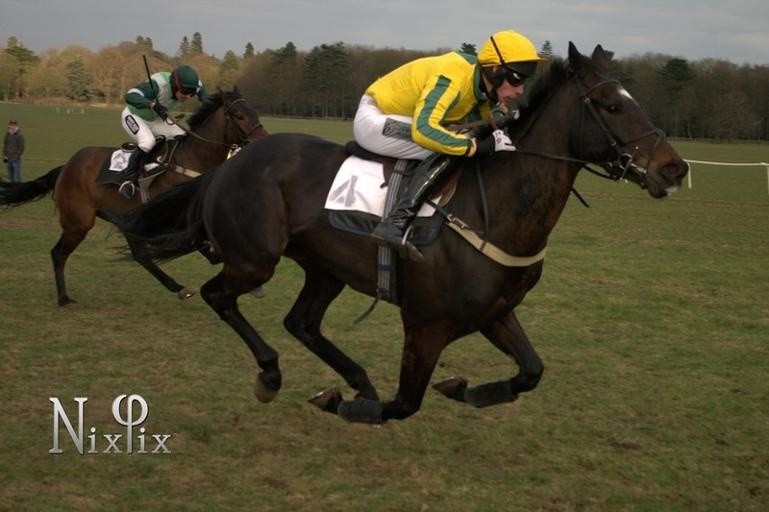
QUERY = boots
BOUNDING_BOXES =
[369,152,460,263]
[118,146,146,200]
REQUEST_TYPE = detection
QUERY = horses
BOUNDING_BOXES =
[0,83,271,310]
[103,40,689,427]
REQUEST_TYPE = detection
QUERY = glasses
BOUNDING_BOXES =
[178,87,196,97]
[502,65,529,87]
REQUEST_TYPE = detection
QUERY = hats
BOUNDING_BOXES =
[476,30,549,68]
[174,63,200,88]
[8,120,17,129]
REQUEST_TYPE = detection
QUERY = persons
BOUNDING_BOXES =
[353,30,548,264]
[3,120,24,183]
[118,65,209,201]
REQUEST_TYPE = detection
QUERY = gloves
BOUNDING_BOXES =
[473,130,516,156]
[153,103,168,120]
